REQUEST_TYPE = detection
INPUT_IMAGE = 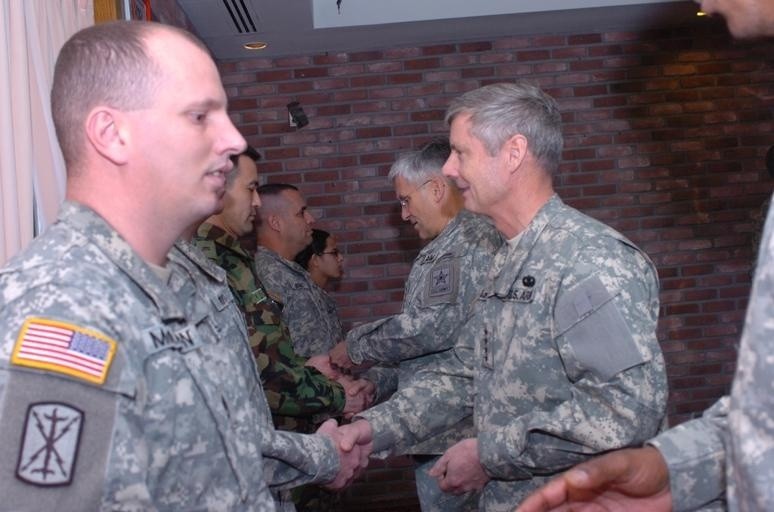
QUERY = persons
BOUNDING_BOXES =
[188,143,375,512]
[1,21,298,512]
[329,136,509,512]
[507,2,773,512]
[330,75,671,512]
[254,181,343,365]
[164,181,366,512]
[344,360,397,423]
[291,227,346,345]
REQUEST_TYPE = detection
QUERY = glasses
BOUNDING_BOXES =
[400,179,434,207]
[315,249,340,257]
